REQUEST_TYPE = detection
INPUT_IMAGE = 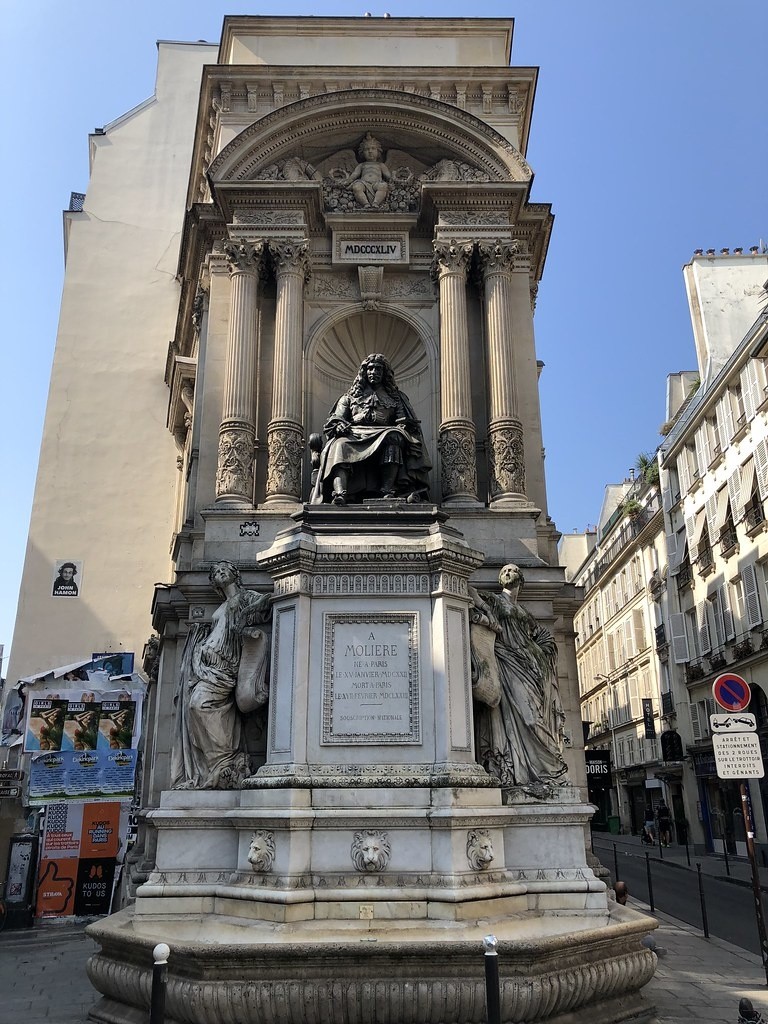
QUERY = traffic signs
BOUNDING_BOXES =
[0,770,24,782]
[0,786,21,798]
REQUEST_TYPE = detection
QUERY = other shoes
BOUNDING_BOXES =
[652,840,655,846]
[662,844,672,848]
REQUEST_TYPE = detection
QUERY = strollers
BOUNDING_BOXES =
[641,819,655,844]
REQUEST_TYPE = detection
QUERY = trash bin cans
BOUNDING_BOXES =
[608,815,620,835]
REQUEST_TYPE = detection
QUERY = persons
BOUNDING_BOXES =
[109,709,130,748]
[40,707,63,751]
[74,709,97,750]
[52,562,78,596]
[643,798,672,848]
[468,563,567,783]
[344,130,392,209]
[172,560,273,789]
[322,354,432,503]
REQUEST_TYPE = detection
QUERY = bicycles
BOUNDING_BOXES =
[0,881,8,931]
